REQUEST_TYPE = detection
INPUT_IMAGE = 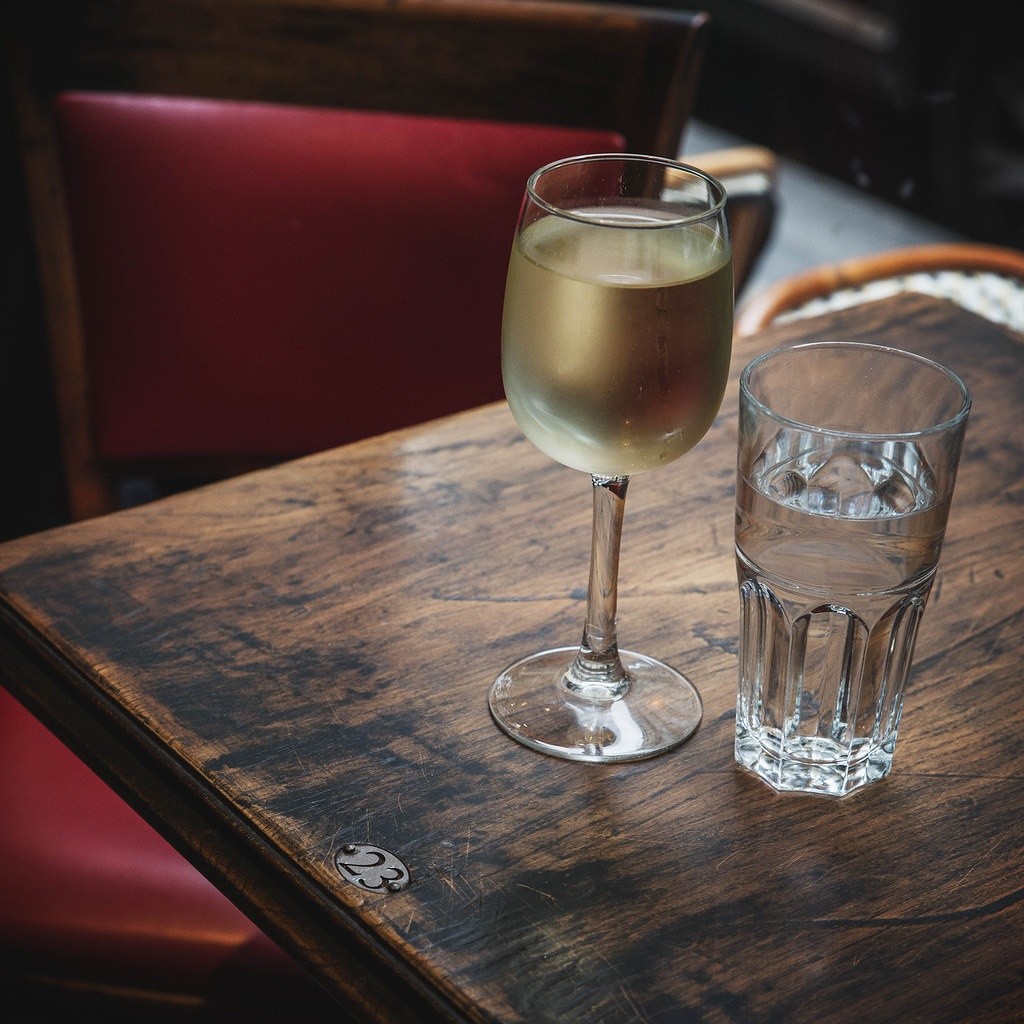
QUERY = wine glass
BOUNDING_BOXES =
[487,153,735,762]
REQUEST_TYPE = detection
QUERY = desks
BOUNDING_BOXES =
[1,283,1024,1024]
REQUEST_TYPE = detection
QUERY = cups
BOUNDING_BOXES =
[734,341,972,797]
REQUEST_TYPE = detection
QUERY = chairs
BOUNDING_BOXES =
[0,0,712,1024]
